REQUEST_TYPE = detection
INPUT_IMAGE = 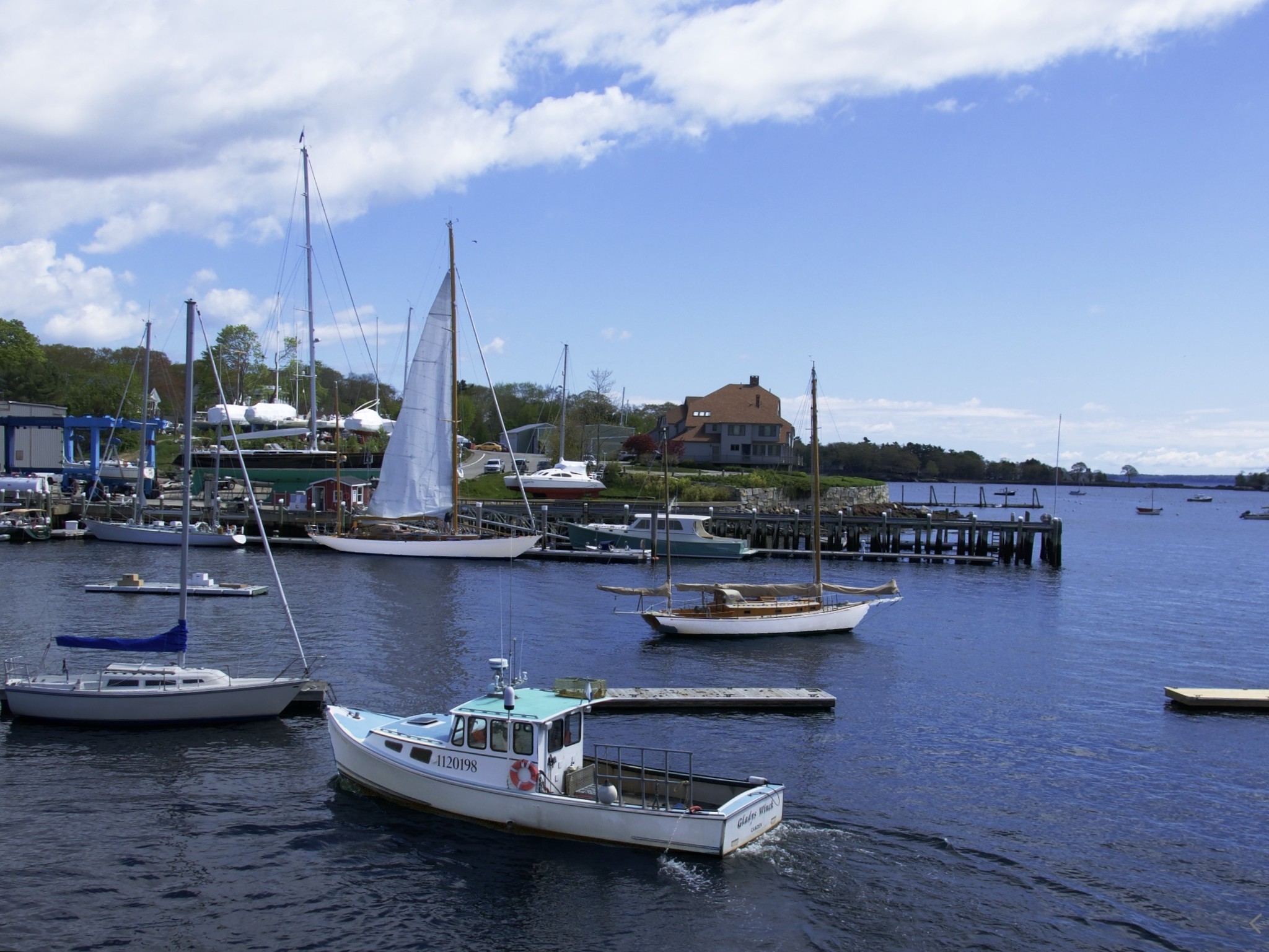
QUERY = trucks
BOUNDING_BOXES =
[618,451,638,460]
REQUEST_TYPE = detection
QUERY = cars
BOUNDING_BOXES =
[537,461,553,472]
[474,442,502,452]
[582,454,597,465]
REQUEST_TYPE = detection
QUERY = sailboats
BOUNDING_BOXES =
[0,127,397,542]
[302,216,544,562]
[3,295,331,732]
[503,343,607,500]
[1069,471,1087,495]
[79,301,248,548]
[594,355,906,639]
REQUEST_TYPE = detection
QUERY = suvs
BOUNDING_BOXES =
[511,459,530,472]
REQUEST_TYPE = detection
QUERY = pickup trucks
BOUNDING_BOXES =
[483,458,505,474]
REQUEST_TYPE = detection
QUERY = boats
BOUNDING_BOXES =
[1187,494,1212,502]
[323,536,785,859]
[1239,506,1269,520]
[994,489,1015,496]
[1136,489,1164,515]
[558,496,759,559]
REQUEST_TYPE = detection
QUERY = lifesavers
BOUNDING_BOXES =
[510,760,538,791]
